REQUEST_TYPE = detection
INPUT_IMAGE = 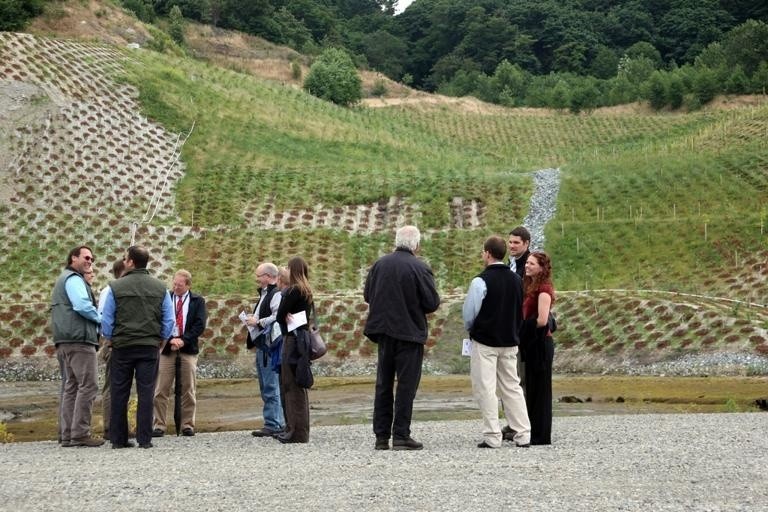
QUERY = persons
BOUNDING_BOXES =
[276,256,327,444]
[98,259,137,440]
[101,245,176,449]
[239,262,284,436]
[57,265,102,443]
[463,236,531,447]
[520,251,557,445]
[363,226,441,451]
[265,267,290,438]
[500,227,531,442]
[153,268,205,437]
[50,246,106,447]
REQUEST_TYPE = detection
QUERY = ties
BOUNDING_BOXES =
[176,296,183,336]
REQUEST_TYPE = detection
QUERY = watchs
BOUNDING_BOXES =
[257,320,261,329]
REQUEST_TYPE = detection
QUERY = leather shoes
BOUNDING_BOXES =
[375,440,389,450]
[393,434,423,450]
[273,428,290,439]
[183,428,194,436]
[278,430,309,443]
[70,433,105,446]
[252,427,283,437]
[150,428,165,438]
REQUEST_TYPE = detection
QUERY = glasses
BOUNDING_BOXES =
[84,256,94,263]
[480,249,488,255]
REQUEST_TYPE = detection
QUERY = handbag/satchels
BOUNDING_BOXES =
[308,299,327,359]
